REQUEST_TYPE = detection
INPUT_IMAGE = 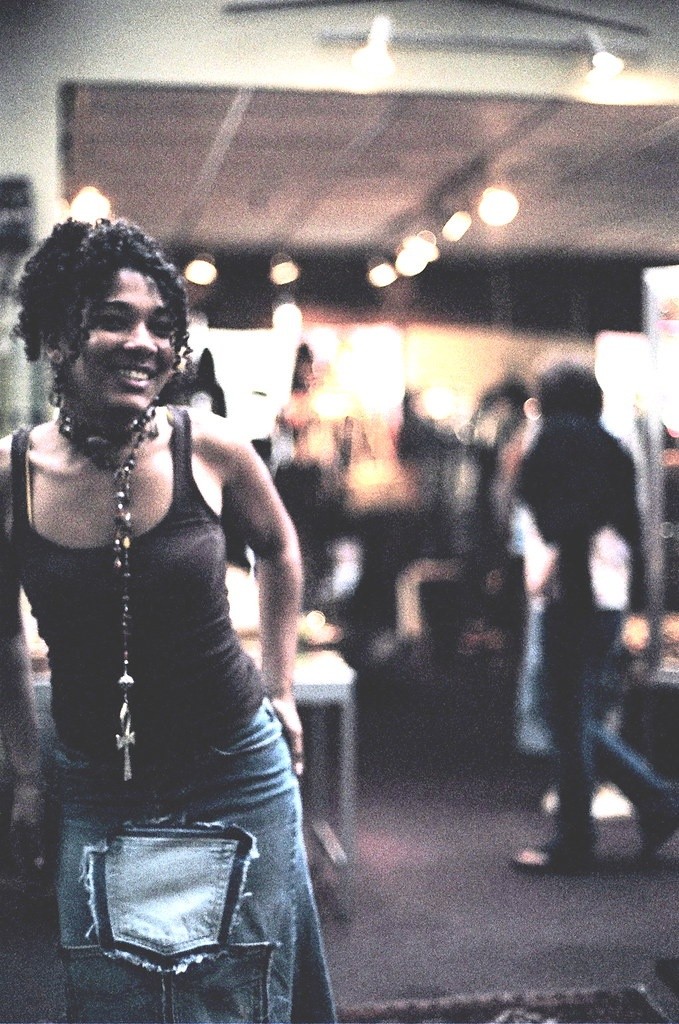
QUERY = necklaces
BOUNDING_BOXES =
[51,395,160,781]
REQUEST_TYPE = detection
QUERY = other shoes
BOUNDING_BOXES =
[636,793,679,860]
[512,831,595,870]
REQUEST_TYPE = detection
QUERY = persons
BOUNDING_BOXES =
[273,358,679,879]
[0,214,337,1023]
